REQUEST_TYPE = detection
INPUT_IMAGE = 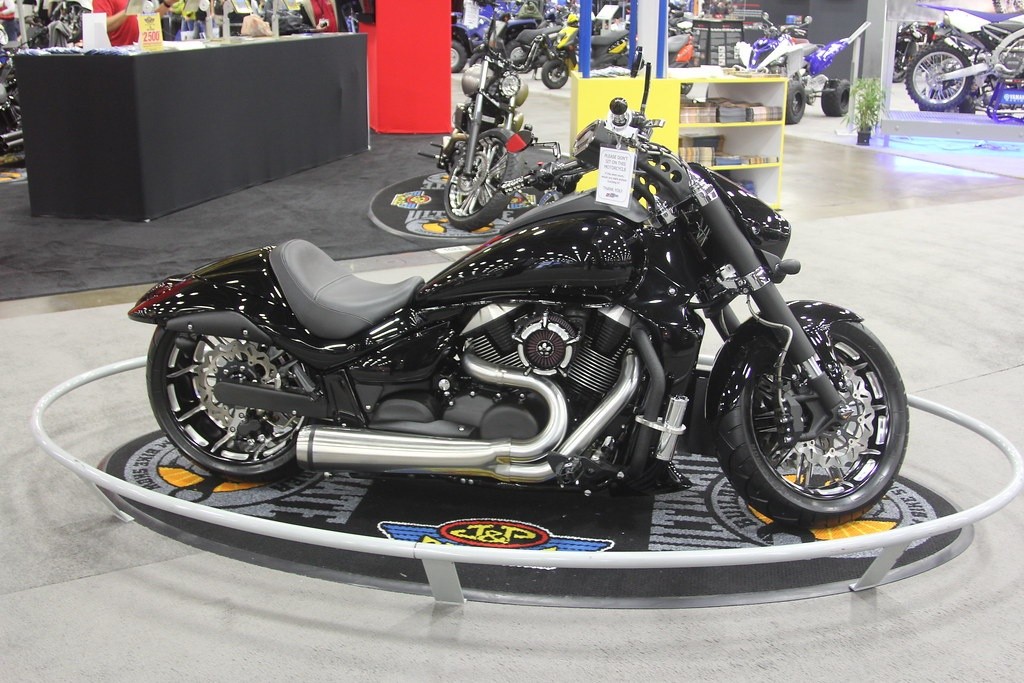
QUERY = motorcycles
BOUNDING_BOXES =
[125,49,913,529]
[0,1,1023,231]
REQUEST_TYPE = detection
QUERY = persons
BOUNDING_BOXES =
[93,0,179,47]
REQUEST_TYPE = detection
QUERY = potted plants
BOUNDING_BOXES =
[839,72,895,147]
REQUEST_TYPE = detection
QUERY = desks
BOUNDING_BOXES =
[11,33,369,223]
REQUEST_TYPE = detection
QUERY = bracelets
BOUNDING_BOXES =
[162,0,170,8]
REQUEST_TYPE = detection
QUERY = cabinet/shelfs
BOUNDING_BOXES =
[570,68,788,211]
